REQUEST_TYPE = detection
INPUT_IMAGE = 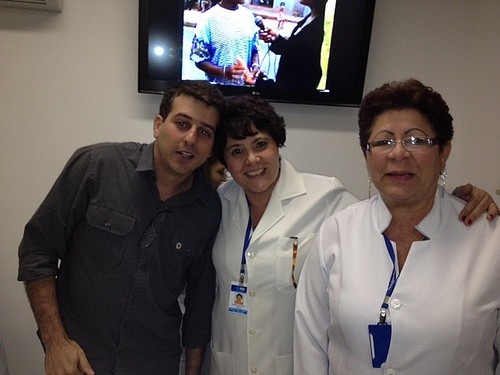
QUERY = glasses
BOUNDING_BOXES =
[366,137,441,152]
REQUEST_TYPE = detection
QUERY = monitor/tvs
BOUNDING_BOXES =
[138,0,376,108]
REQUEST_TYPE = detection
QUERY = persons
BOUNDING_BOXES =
[207,153,226,189]
[200,96,498,375]
[293,78,500,375]
[18,79,226,375]
[189,0,260,85]
[244,0,328,89]
[277,2,285,28]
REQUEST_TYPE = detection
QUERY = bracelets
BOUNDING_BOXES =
[252,63,260,68]
[222,65,227,78]
